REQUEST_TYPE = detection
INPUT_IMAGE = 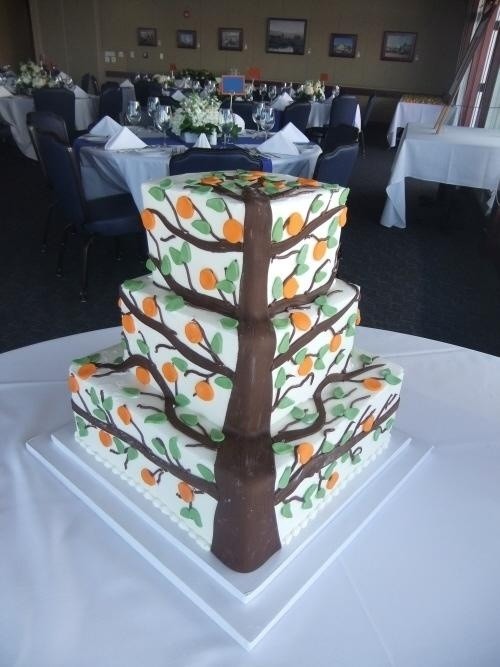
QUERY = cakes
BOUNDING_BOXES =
[65,167,405,574]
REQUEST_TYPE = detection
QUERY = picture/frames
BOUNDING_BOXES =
[176,29,197,49]
[380,31,417,63]
[136,27,157,47]
[329,33,357,58]
[217,27,243,51]
[265,17,307,55]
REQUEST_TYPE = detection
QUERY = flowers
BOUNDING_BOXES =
[14,57,56,89]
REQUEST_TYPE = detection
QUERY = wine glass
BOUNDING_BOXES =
[1,75,75,94]
[126,95,172,150]
[252,100,275,141]
[187,79,341,105]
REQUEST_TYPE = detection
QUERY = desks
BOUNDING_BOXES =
[378,122,500,229]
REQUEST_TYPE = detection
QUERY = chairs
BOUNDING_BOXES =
[2,75,376,172]
[27,110,145,303]
